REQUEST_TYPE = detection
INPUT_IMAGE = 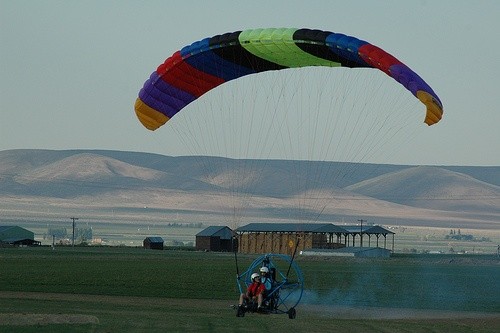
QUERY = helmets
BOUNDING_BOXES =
[251,273,259,281]
[260,266,269,273]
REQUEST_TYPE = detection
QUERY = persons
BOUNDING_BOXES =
[259,266,272,306]
[235,272,266,311]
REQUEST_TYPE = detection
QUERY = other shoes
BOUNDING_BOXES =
[257,307,261,312]
